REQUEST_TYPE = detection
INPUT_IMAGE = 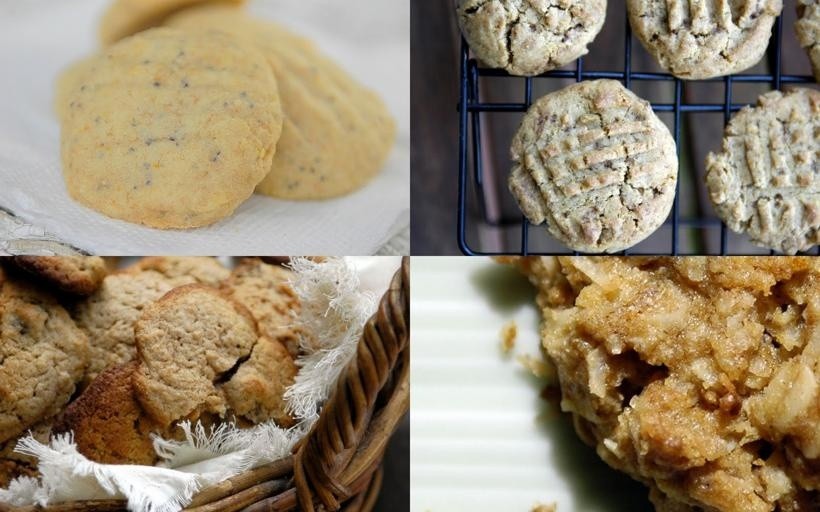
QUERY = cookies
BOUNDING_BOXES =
[457,0,820,255]
[54,0,398,230]
[0,255,337,491]
